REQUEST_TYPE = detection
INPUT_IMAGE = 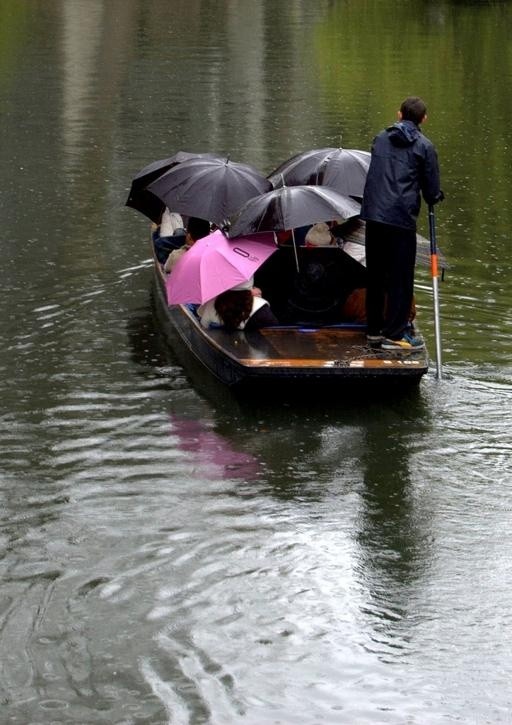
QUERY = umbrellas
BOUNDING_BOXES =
[125,147,374,307]
[341,218,450,270]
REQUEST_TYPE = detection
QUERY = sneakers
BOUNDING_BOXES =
[366,324,426,350]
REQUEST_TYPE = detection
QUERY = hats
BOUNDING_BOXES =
[305,222,331,246]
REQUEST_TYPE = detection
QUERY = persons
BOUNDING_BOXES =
[200,275,278,330]
[360,97,444,348]
[341,288,416,323]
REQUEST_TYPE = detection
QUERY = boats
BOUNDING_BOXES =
[150,214,430,400]
